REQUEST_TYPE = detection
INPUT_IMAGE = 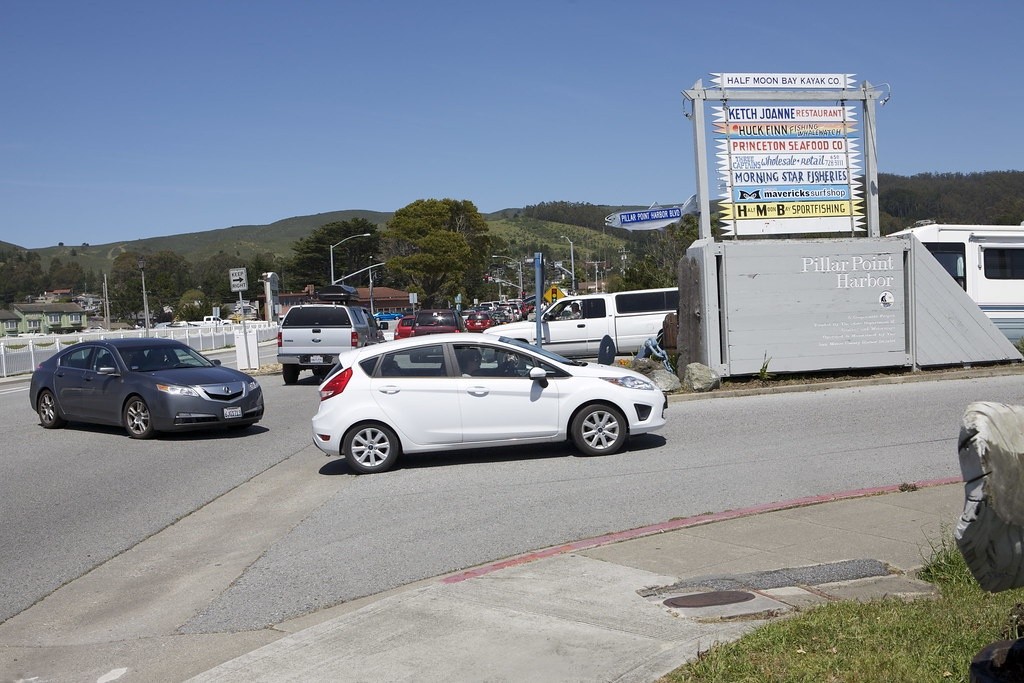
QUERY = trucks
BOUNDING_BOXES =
[886,221,1024,344]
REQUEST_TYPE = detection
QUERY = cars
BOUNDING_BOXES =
[498,306,518,323]
[29,336,265,440]
[400,307,419,315]
[490,310,510,323]
[394,315,415,340]
[462,310,476,321]
[472,296,536,321]
[310,332,669,475]
[81,325,108,332]
[374,312,404,320]
[465,311,497,333]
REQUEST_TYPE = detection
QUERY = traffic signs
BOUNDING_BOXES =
[229,269,247,292]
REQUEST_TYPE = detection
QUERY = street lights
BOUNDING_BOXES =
[561,235,575,296]
[492,255,522,299]
[330,233,370,304]
[138,259,149,338]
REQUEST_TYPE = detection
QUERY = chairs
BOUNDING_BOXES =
[102,353,119,369]
[440,348,462,378]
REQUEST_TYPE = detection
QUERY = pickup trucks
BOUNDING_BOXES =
[187,316,231,326]
[18,330,46,338]
[482,286,680,370]
[276,303,385,384]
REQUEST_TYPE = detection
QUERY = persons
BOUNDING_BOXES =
[461,348,482,378]
[567,303,581,319]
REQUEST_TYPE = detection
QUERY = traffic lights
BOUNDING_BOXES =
[374,276,379,288]
[522,291,525,298]
[484,275,488,283]
[552,288,557,303]
[375,267,382,279]
[550,262,554,270]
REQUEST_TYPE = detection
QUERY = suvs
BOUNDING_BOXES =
[409,309,468,363]
